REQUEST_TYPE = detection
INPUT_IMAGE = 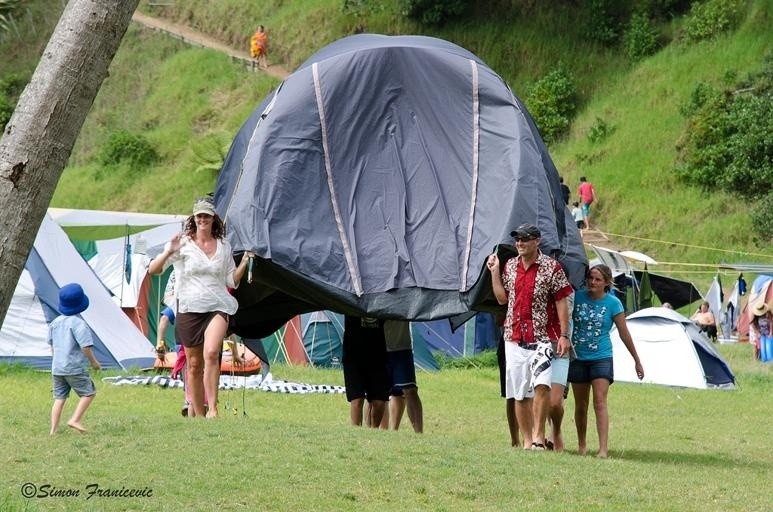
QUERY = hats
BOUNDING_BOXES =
[510,223,540,238]
[192,200,215,216]
[752,302,767,316]
[58,283,88,316]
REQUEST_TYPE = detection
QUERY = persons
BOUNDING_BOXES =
[341,312,393,428]
[567,263,645,460]
[146,197,258,422]
[152,308,246,416]
[253,24,268,69]
[362,396,393,432]
[483,220,577,456]
[46,282,102,438]
[570,202,586,239]
[575,176,599,231]
[381,312,424,435]
[558,176,572,211]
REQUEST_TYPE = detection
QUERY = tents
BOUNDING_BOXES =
[204,31,592,341]
[1,207,771,395]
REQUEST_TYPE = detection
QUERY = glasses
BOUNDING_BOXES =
[514,237,536,242]
